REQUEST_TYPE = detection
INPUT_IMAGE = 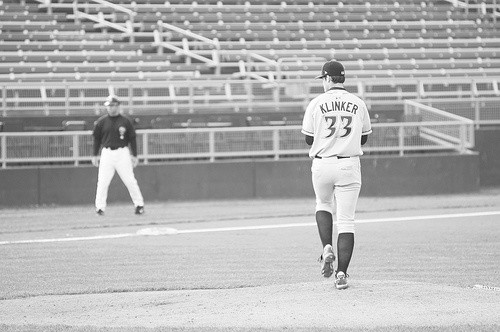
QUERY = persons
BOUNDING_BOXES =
[91,95,145,214]
[301,60,372,289]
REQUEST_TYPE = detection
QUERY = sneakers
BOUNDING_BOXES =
[335,271,348,289]
[319,244,335,279]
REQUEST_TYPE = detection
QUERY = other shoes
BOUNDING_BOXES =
[96,210,104,216]
[136,206,144,214]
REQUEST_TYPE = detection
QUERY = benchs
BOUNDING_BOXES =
[0,0,500,112]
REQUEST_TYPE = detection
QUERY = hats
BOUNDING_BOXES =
[315,61,345,78]
[104,96,119,106]
